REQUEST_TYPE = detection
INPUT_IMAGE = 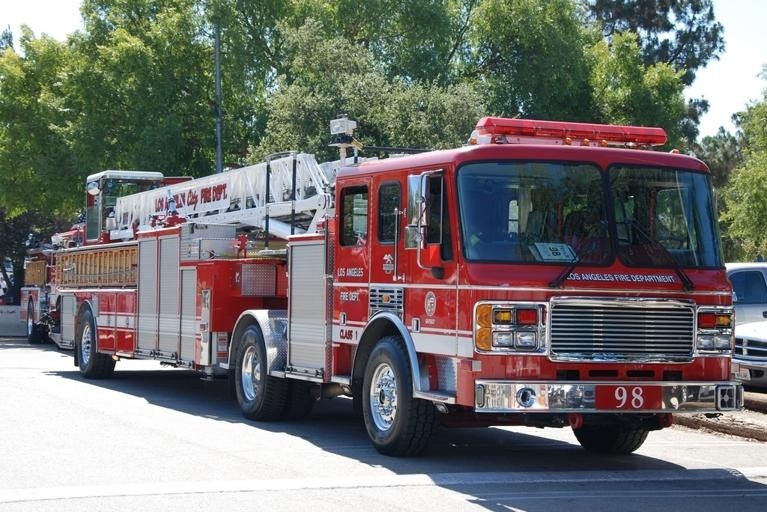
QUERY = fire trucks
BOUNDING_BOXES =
[47,111,745,458]
[20,168,196,345]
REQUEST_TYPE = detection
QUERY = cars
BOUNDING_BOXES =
[722,261,767,395]
